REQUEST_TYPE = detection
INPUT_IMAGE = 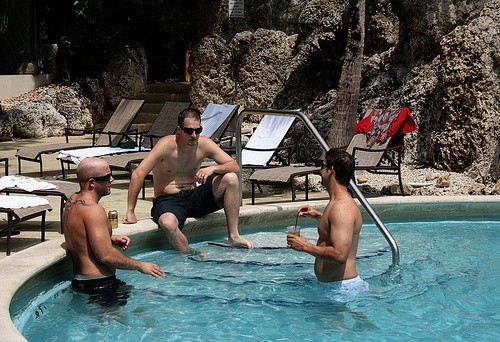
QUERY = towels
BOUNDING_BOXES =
[196,101,238,139]
[0,174,59,194]
[56,145,153,167]
[0,195,50,211]
[236,107,301,167]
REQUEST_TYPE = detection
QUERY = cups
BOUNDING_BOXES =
[287,226,301,237]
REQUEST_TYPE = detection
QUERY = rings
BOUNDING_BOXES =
[202,175,205,177]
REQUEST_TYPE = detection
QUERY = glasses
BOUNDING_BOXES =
[85,168,113,182]
[320,162,333,170]
[178,124,203,134]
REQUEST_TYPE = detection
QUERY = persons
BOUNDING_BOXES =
[287,148,381,332]
[123,108,252,261]
[64,158,166,326]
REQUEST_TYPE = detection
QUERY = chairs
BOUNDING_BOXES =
[0,158,81,256]
[57,100,193,180]
[242,108,301,206]
[14,97,145,177]
[249,107,417,203]
[141,103,240,200]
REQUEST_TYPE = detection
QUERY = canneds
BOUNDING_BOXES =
[109,210,118,228]
